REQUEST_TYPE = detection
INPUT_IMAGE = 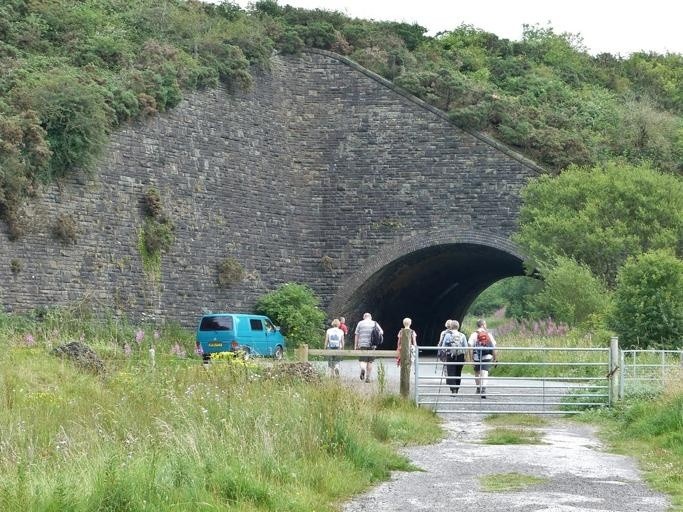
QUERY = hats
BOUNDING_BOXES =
[331,319,340,326]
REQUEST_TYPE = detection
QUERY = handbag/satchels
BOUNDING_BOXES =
[372,329,384,344]
[438,349,445,359]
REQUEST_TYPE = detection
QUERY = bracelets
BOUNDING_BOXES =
[324,345,327,346]
[494,358,496,360]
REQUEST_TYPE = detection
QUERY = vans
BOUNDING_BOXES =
[196,314,287,364]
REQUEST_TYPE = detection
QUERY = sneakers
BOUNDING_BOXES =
[366,378,371,383]
[360,370,365,380]
[331,368,340,379]
[452,390,458,393]
[477,390,485,398]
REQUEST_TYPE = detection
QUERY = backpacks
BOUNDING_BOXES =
[451,335,465,356]
[329,332,340,348]
[473,331,494,361]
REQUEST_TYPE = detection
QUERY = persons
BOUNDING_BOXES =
[340,317,349,335]
[396,317,417,393]
[265,320,272,333]
[324,318,344,378]
[354,312,383,382]
[469,317,499,401]
[436,318,452,385]
[441,319,468,396]
